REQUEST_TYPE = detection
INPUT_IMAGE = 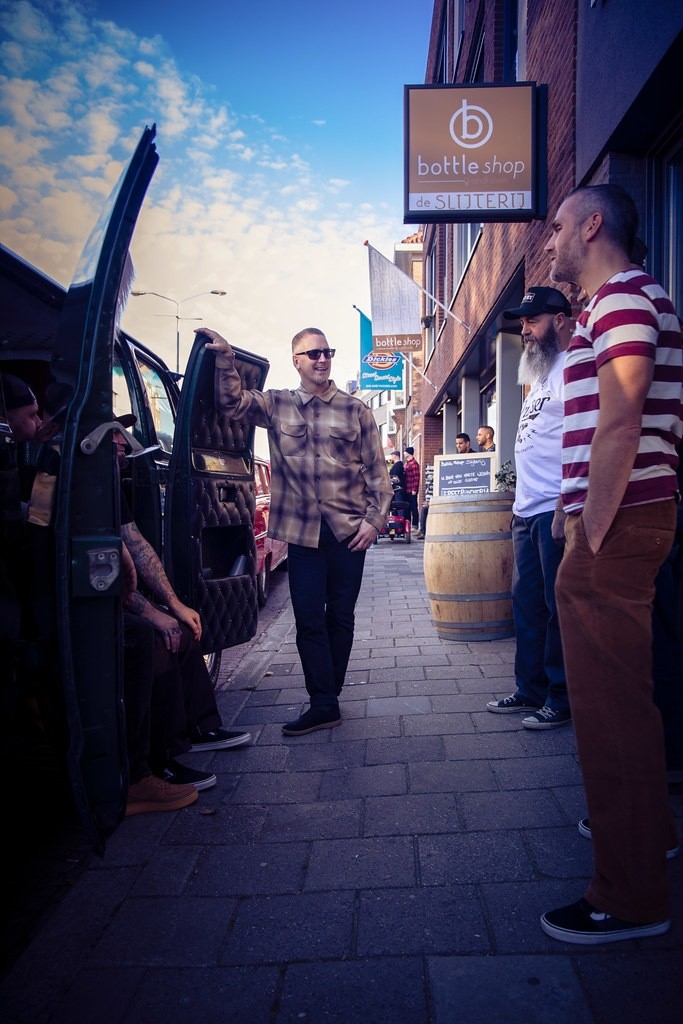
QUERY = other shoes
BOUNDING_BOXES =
[412,532,421,536]
[189,729,252,751]
[152,762,217,790]
[416,536,425,539]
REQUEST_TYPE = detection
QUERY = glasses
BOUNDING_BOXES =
[295,349,336,360]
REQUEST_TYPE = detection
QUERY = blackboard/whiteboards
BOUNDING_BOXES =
[433,451,500,496]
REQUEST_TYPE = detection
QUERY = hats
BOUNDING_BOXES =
[503,286,572,320]
[0,372,37,410]
[405,447,413,455]
[113,412,136,430]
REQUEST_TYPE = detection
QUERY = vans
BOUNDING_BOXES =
[0,242,271,833]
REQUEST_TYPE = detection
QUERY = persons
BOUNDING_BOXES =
[193,327,393,736]
[455,433,477,453]
[402,447,420,531]
[390,451,405,516]
[412,501,429,539]
[539,183,682,945]
[0,376,250,814]
[486,286,571,730]
[476,426,495,451]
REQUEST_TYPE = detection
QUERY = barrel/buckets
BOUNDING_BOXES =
[422,491,517,642]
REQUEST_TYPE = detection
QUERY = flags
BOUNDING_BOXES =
[368,247,422,354]
[360,312,402,391]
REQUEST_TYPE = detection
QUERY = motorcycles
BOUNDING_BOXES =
[373,475,411,544]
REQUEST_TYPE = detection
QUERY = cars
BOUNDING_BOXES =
[251,453,287,607]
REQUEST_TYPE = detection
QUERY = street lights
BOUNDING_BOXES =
[131,289,226,378]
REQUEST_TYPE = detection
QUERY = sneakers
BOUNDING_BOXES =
[522,706,572,729]
[540,898,672,944]
[487,689,542,712]
[579,818,679,859]
[282,704,341,734]
[124,776,198,816]
